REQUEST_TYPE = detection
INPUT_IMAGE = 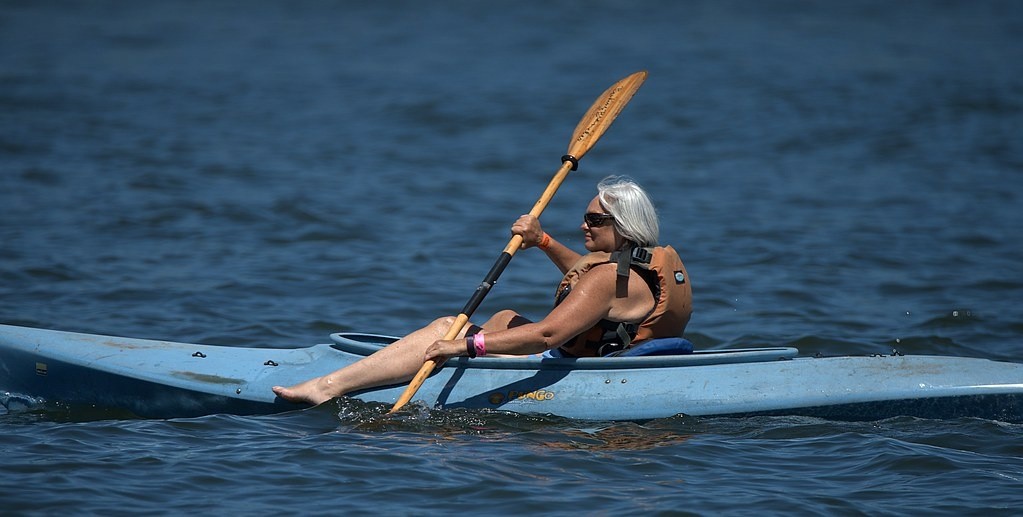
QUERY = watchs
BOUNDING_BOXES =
[466,333,476,359]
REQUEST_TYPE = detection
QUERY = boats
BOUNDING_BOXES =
[0,324,1023,421]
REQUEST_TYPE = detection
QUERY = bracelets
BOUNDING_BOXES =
[475,334,486,356]
[537,231,552,251]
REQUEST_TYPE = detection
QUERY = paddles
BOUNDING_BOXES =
[387,68,648,415]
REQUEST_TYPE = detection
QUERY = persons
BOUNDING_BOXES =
[272,175,694,406]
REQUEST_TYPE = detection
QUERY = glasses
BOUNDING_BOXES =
[584,213,616,227]
